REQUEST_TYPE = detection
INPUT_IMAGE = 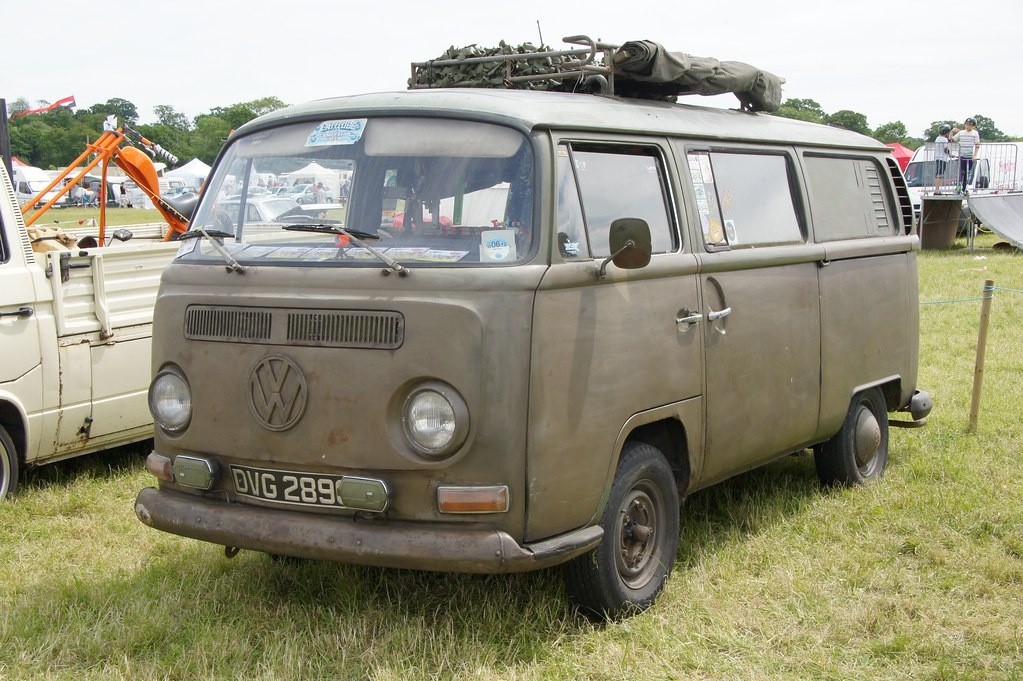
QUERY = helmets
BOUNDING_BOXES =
[938,124,951,135]
[964,117,977,126]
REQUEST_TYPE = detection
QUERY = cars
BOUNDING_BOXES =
[158,176,333,224]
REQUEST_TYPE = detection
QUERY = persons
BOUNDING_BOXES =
[257,175,311,188]
[119,182,130,208]
[949,117,980,194]
[313,183,327,216]
[98,184,102,208]
[934,124,955,195]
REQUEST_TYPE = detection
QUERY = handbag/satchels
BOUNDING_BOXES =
[975,159,990,188]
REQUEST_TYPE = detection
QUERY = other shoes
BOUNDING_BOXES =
[934,192,942,196]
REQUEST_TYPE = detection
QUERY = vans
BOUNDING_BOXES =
[131,35,934,622]
[11,166,66,210]
[903,141,1022,234]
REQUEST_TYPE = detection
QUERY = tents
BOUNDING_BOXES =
[291,162,340,204]
[163,159,225,199]
[886,142,916,179]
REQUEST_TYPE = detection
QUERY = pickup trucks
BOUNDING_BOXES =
[0,155,344,502]
